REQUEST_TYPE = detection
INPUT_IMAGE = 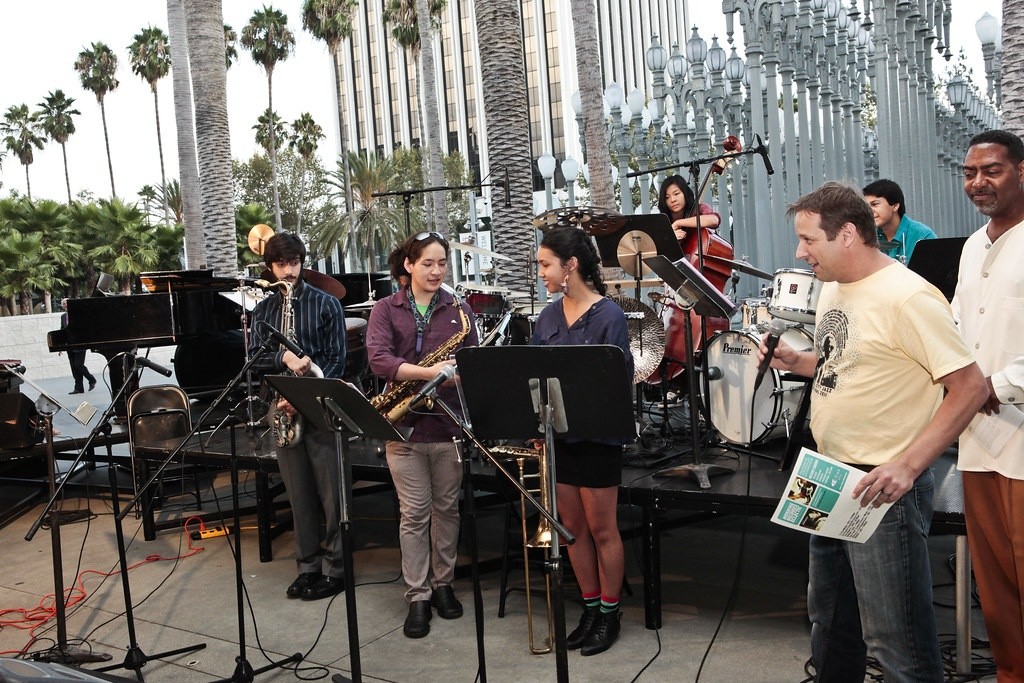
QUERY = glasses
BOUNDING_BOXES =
[408,231,445,257]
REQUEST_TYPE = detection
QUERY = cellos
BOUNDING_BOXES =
[643,133,747,386]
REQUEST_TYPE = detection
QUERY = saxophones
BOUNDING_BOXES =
[252,277,326,452]
[369,292,471,425]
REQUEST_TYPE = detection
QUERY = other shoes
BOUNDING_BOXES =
[69,388,85,394]
[88,379,97,391]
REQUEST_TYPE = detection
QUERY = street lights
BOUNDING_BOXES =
[570,1,1007,316]
[535,151,557,303]
[551,155,580,303]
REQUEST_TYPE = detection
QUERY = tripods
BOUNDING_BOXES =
[3,333,306,683]
[597,213,782,488]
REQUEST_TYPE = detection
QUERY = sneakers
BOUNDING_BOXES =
[566,599,602,649]
[579,609,623,657]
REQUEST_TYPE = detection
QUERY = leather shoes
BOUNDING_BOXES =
[431,584,463,620]
[302,575,345,600]
[286,571,322,598]
[404,599,433,638]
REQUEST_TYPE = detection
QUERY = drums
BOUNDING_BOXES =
[696,322,815,447]
[767,266,823,326]
[740,296,801,330]
[459,282,509,319]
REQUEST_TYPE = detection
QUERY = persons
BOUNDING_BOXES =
[863,180,937,267]
[658,175,721,408]
[366,229,479,638]
[61,297,97,394]
[756,178,990,683]
[529,229,634,656]
[951,129,1024,683]
[250,233,349,601]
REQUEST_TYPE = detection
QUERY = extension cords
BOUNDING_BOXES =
[191,526,235,540]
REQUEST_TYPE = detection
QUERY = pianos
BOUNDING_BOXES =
[46,268,253,416]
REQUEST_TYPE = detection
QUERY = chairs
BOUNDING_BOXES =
[907,237,969,304]
[127,385,201,520]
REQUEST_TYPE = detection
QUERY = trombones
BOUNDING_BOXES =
[515,440,569,656]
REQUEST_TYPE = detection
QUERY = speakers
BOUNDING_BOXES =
[0,656,140,683]
[0,392,46,449]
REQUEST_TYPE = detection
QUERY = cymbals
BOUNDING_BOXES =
[478,267,512,275]
[448,239,517,263]
[617,230,658,278]
[879,240,901,249]
[695,252,774,281]
[248,224,274,255]
[260,267,347,300]
[532,205,627,237]
[528,242,535,280]
[343,298,376,312]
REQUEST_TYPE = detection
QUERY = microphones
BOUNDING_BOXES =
[264,321,305,359]
[757,136,774,174]
[754,318,786,390]
[707,367,724,381]
[408,364,456,407]
[505,168,511,208]
[129,352,172,378]
